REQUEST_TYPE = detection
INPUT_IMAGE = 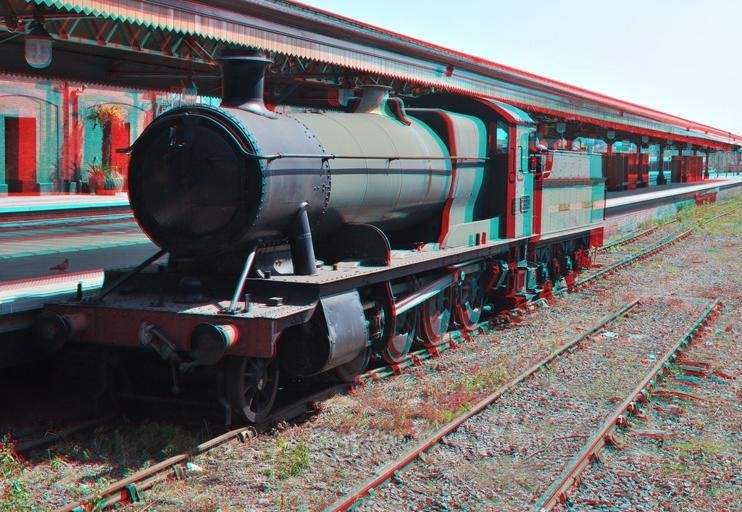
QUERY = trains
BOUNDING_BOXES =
[31,53,606,434]
